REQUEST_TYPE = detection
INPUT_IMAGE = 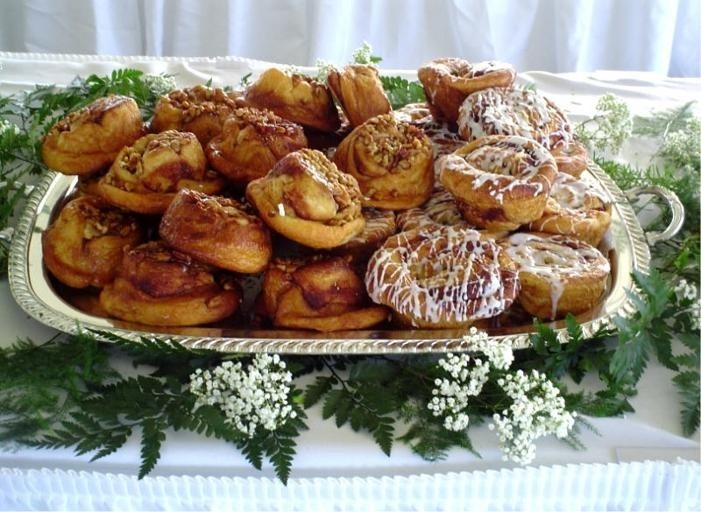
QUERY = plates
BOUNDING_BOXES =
[1,149,684,357]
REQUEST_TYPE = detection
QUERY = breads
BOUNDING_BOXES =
[525,172,612,249]
[330,114,435,211]
[274,208,396,258]
[242,68,341,135]
[457,86,568,146]
[159,188,271,275]
[205,103,307,181]
[255,256,387,332]
[364,224,520,331]
[40,93,142,175]
[499,230,610,322]
[99,242,242,327]
[98,128,221,214]
[246,147,365,251]
[440,135,557,233]
[396,191,507,240]
[150,84,241,145]
[327,62,391,126]
[41,195,139,289]
[418,58,510,121]
